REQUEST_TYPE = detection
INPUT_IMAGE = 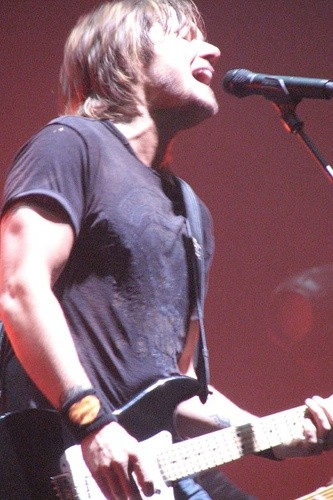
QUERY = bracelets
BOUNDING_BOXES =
[59,388,119,445]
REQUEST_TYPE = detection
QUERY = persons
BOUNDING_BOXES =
[0,0,333,500]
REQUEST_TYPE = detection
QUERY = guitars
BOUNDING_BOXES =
[0,375,333,500]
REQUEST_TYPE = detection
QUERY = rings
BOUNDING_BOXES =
[318,438,328,444]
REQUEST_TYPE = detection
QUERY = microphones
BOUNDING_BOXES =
[222,68,333,98]
